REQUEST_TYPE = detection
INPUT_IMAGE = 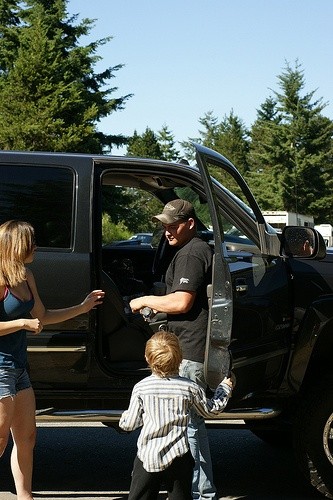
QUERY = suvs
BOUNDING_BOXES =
[0,141,333,491]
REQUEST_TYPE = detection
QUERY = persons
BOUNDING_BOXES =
[129,199,217,500]
[119,330,237,500]
[0,219,104,500]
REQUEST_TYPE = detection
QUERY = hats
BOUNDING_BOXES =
[151,199,195,225]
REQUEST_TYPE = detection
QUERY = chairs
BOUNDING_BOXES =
[100,269,167,329]
